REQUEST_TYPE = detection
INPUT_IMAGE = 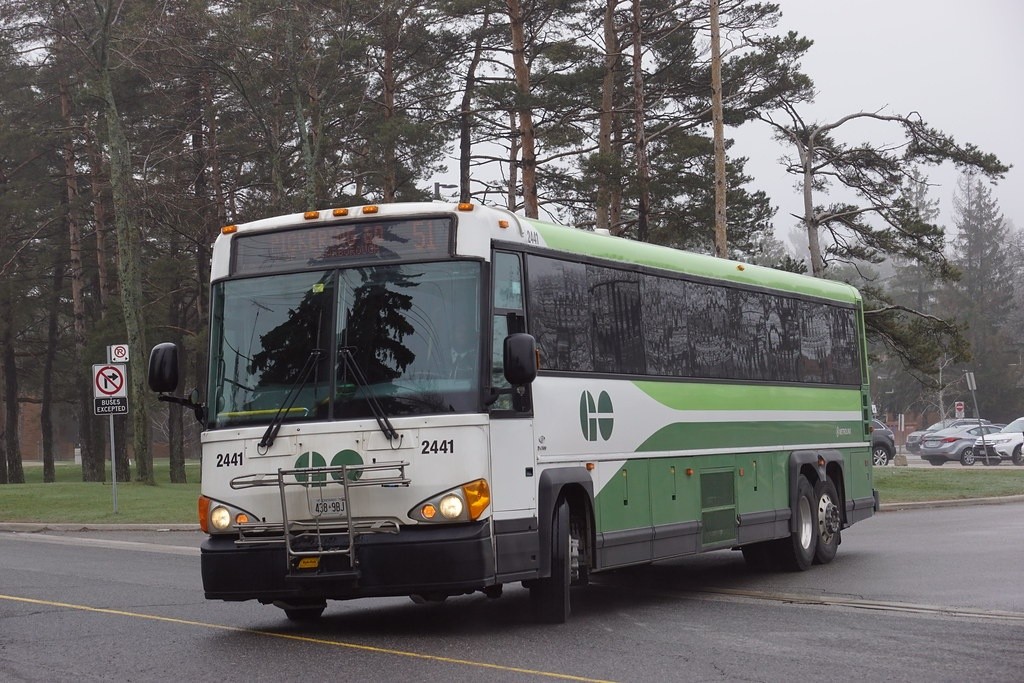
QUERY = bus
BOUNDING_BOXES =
[149,202,879,624]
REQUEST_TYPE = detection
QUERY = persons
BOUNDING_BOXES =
[436,312,481,380]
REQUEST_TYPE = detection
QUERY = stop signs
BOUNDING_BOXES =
[956,402,964,411]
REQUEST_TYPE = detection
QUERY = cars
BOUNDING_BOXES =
[906,418,1007,466]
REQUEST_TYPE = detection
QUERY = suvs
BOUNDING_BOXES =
[872,419,896,467]
[972,417,1024,466]
